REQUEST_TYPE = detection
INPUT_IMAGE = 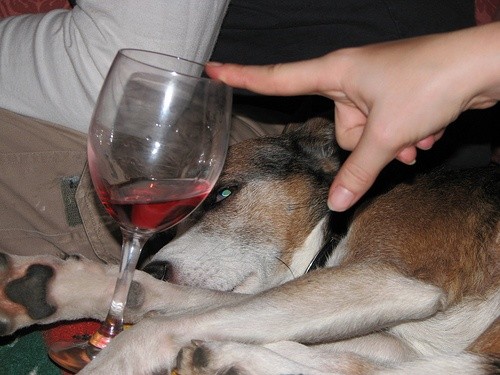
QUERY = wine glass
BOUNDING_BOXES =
[47,48,232,373]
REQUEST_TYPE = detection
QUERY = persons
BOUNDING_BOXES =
[0,0,500,269]
[206,19,500,212]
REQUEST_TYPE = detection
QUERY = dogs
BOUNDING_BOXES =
[0,117,500,375]
